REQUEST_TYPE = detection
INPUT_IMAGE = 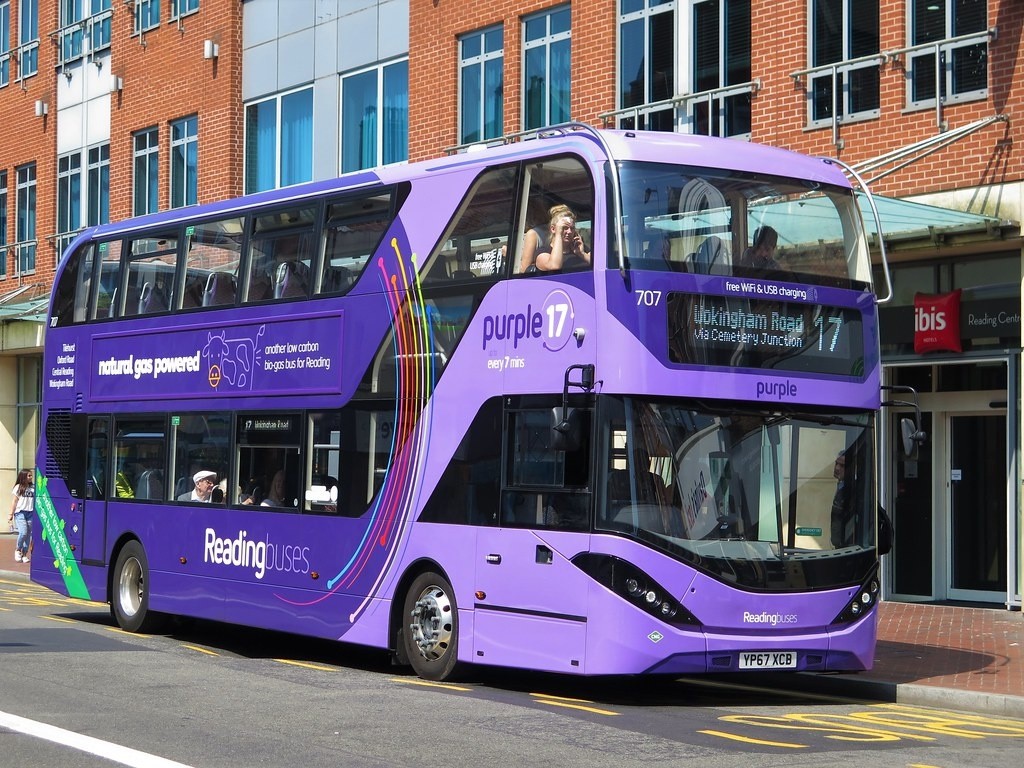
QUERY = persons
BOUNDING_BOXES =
[7,468,36,563]
[143,274,174,313]
[501,204,591,273]
[607,448,669,502]
[830,454,857,674]
[262,469,288,507]
[177,468,258,505]
[116,455,149,499]
[320,475,339,512]
[742,226,785,271]
[645,236,673,259]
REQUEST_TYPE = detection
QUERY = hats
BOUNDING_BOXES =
[192,470,217,485]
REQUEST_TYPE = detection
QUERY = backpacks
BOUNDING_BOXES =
[876,504,894,555]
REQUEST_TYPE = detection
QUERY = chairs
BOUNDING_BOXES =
[95,451,718,541]
[108,254,477,319]
[692,236,731,275]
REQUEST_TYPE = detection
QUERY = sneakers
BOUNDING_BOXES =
[15,549,23,563]
[22,556,30,563]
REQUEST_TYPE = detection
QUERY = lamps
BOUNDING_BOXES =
[35,100,48,117]
[110,74,123,92]
[204,40,219,59]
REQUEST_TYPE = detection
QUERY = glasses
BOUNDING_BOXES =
[200,479,216,484]
[28,474,32,477]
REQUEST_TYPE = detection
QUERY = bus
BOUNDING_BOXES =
[30,122,925,684]
